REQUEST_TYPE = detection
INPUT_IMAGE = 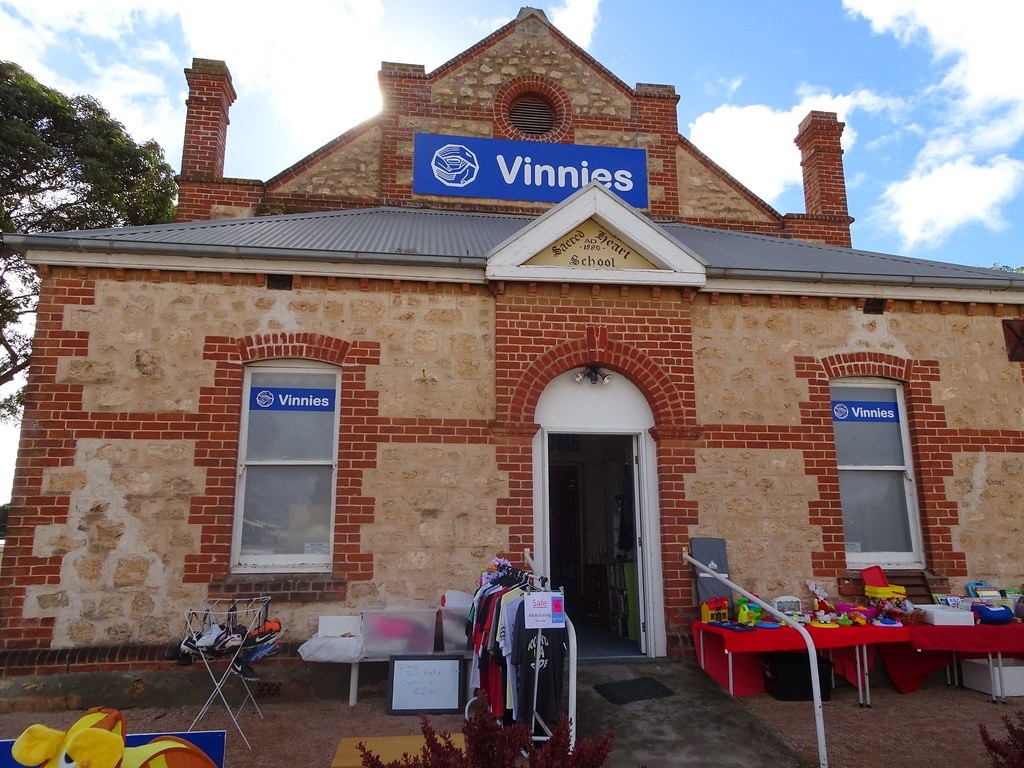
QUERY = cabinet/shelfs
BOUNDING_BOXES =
[584,564,609,626]
[606,563,637,641]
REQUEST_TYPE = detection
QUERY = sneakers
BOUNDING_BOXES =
[163,597,285,682]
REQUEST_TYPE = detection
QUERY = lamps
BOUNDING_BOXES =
[573,367,612,385]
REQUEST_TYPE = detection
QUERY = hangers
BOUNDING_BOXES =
[489,563,541,598]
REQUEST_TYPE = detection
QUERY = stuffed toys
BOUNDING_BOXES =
[11,705,218,768]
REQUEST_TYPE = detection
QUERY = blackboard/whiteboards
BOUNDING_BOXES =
[386,653,465,716]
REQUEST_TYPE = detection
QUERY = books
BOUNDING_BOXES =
[932,587,1024,614]
[608,564,631,641]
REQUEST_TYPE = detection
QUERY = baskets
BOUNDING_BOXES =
[884,608,926,624]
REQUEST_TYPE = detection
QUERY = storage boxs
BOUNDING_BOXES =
[961,658,1024,697]
[762,654,834,701]
[438,604,474,654]
[914,603,974,625]
[361,605,438,656]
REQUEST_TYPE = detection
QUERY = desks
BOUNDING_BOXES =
[693,616,910,708]
[879,614,1024,705]
